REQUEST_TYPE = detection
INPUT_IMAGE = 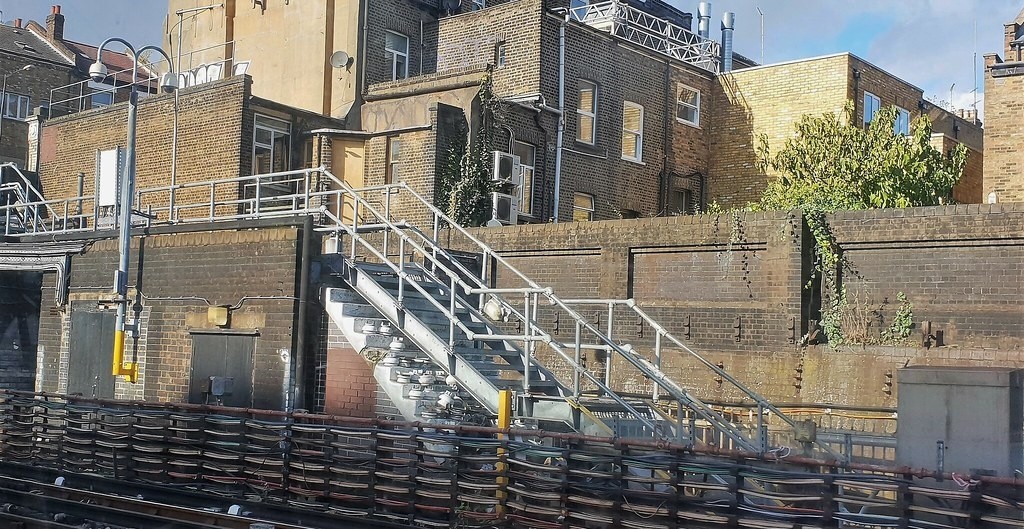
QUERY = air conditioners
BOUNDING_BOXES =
[489,151,520,187]
[488,192,517,225]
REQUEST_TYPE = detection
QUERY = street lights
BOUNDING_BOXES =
[88,38,178,385]
